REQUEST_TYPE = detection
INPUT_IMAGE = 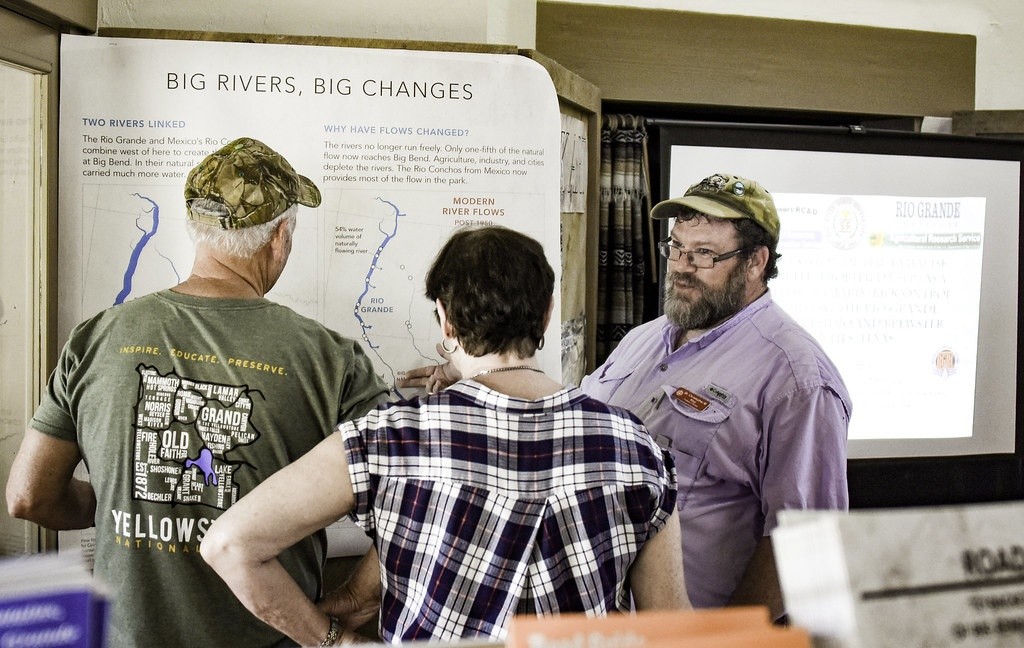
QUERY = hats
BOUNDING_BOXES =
[650,173,780,240]
[185,138,322,230]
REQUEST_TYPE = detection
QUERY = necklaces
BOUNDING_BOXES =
[467,363,545,381]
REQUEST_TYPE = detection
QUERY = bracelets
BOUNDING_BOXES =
[322,614,340,648]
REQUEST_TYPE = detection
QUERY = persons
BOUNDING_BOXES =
[397,173,851,616]
[6,139,396,648]
[199,225,693,648]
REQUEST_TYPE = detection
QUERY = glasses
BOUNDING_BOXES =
[658,236,757,269]
